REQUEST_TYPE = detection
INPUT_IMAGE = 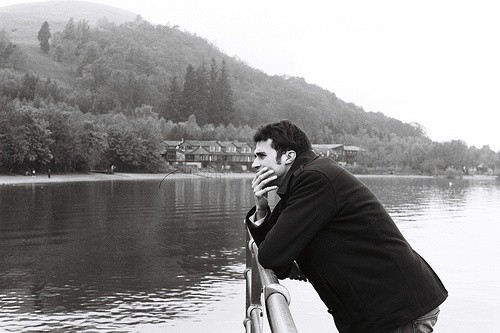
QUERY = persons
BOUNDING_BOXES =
[32,169,36,177]
[48,169,52,178]
[111,164,116,172]
[244,119,448,333]
